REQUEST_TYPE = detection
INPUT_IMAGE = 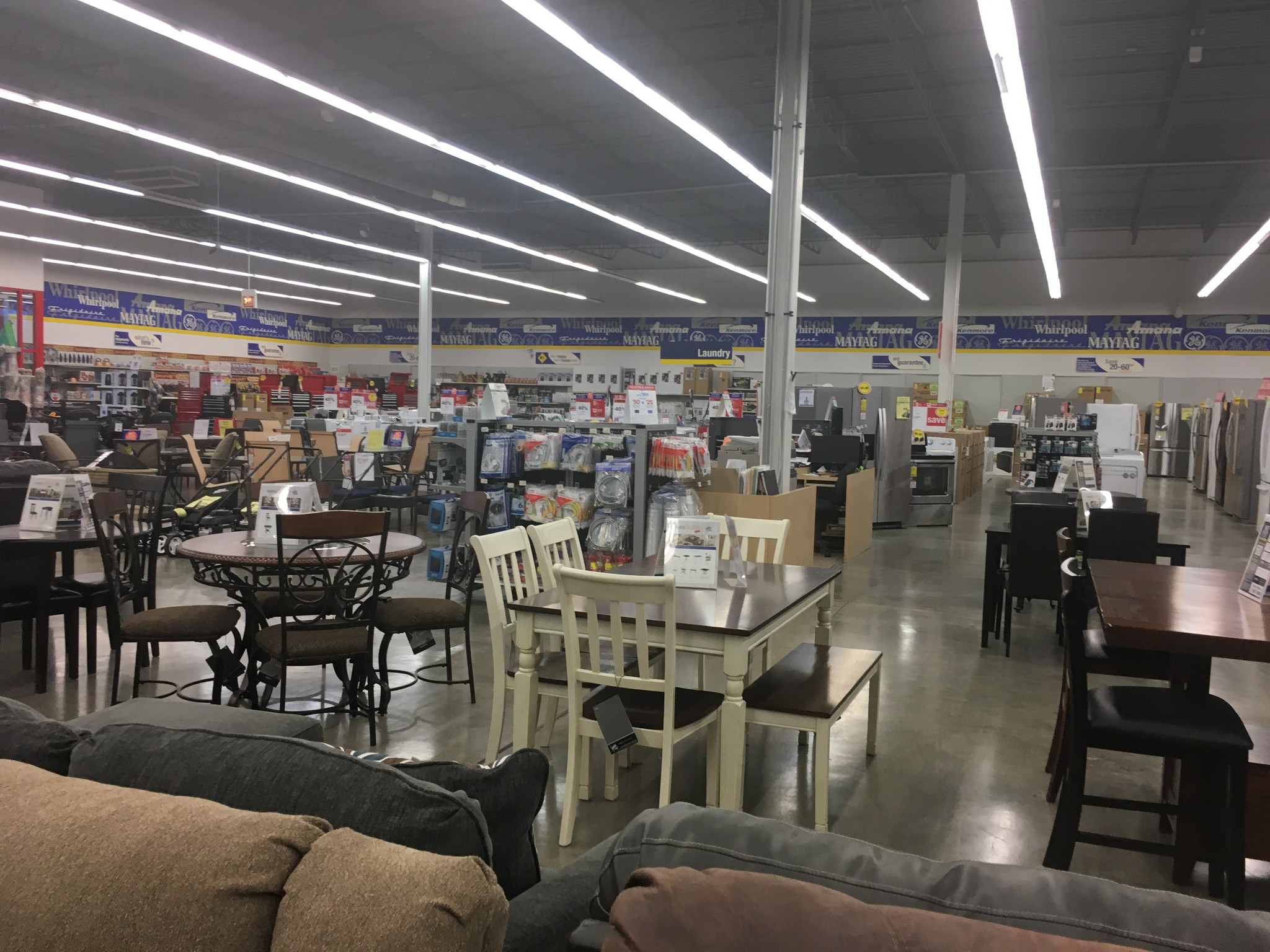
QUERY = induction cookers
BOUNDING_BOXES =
[911,433,955,459]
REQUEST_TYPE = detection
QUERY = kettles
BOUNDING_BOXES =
[131,392,138,404]
[68,377,77,382]
[103,358,113,366]
[105,374,111,385]
[131,374,138,386]
[118,373,126,386]
[117,392,124,404]
[95,358,103,366]
[105,393,112,404]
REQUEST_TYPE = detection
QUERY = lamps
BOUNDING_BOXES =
[0,0,1270,308]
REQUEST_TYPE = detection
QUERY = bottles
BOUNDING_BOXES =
[1064,437,1079,454]
[1038,436,1051,453]
[1050,436,1063,453]
[1081,438,1094,454]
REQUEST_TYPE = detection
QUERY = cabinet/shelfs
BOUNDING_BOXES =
[37,345,149,419]
[427,409,478,602]
[438,382,570,407]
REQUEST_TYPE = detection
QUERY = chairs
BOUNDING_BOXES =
[0,416,1255,908]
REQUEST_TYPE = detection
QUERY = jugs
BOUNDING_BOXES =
[451,371,506,383]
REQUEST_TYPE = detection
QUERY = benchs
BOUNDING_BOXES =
[738,642,885,830]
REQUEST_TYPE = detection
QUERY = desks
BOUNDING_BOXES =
[0,517,156,694]
[0,440,43,460]
[506,557,838,805]
[171,529,427,747]
[1082,552,1270,665]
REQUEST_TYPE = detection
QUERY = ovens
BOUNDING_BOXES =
[909,459,956,504]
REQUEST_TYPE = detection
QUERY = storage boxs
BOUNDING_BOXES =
[529,367,732,422]
[235,391,254,411]
[254,393,268,411]
[231,410,283,429]
[269,404,293,427]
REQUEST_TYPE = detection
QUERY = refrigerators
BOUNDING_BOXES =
[1029,396,1086,428]
[1187,407,1213,494]
[1086,403,1141,451]
[1207,402,1231,506]
[1252,402,1270,533]
[1223,400,1266,525]
[1145,402,1191,478]
[850,386,914,530]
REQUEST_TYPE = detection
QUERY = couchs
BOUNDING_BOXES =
[0,690,1270,952]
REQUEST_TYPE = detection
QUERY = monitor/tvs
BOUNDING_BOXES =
[808,436,861,472]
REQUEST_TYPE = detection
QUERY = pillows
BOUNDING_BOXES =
[307,731,549,900]
